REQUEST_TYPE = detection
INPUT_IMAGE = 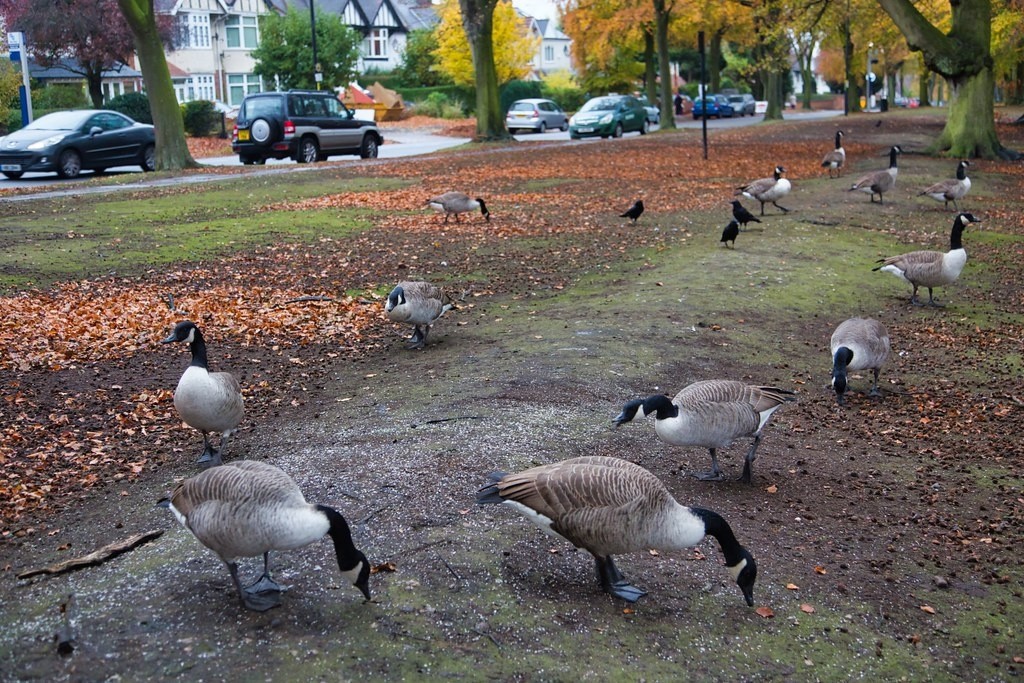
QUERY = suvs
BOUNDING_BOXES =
[231,87,384,167]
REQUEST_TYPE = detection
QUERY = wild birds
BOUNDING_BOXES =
[421,191,491,228]
[156,458,373,613]
[384,281,468,351]
[870,212,982,309]
[874,119,883,129]
[728,199,763,232]
[845,145,907,206]
[733,165,793,217]
[915,160,972,214]
[829,316,891,407]
[611,378,799,488]
[820,130,846,179]
[617,199,644,227]
[474,455,759,607]
[160,320,247,468]
[719,220,740,249]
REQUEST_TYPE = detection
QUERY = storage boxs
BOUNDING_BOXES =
[340,81,405,122]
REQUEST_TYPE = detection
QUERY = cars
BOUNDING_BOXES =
[506,98,568,134]
[0,109,159,180]
[692,94,734,119]
[638,97,661,126]
[568,94,651,141]
[726,94,756,117]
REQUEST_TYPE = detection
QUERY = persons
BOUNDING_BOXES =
[674,94,683,115]
